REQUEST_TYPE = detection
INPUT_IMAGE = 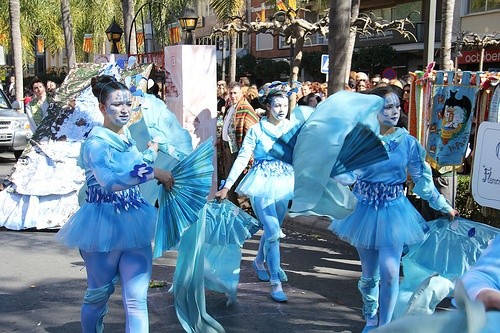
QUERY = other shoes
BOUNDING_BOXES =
[253,260,270,281]
[271,290,288,301]
[362,323,378,333]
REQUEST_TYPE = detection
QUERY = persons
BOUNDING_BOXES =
[215,75,500,333]
[53,74,175,333]
[6,75,60,133]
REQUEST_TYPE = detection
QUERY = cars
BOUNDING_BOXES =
[0,88,33,161]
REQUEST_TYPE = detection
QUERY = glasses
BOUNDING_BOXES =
[404,90,410,94]
